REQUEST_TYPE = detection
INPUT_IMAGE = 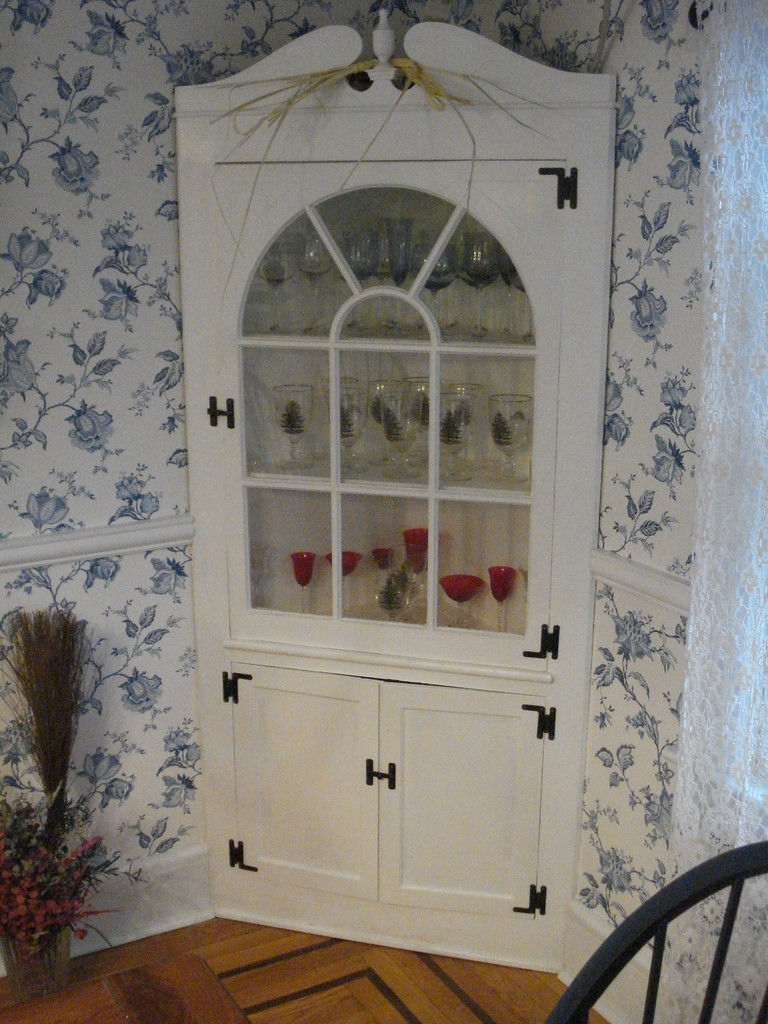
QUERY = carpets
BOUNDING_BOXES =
[0,954,252,1024]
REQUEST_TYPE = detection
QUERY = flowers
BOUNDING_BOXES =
[0,822,123,941]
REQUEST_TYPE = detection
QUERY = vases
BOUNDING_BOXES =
[1,937,55,1004]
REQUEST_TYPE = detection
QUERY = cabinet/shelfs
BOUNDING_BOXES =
[166,22,614,975]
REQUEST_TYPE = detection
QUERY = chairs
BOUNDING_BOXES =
[546,839,768,1024]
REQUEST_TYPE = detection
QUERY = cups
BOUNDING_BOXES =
[372,549,392,569]
[325,377,358,413]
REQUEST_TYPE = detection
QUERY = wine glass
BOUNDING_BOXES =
[374,564,414,622]
[404,528,428,607]
[302,232,336,336]
[449,384,484,470]
[439,575,485,629]
[255,241,301,333]
[489,393,533,490]
[488,567,514,633]
[326,551,363,617]
[380,391,424,479]
[272,385,315,470]
[328,387,368,474]
[368,380,409,465]
[403,377,444,465]
[439,392,477,486]
[337,216,535,344]
[291,552,315,614]
